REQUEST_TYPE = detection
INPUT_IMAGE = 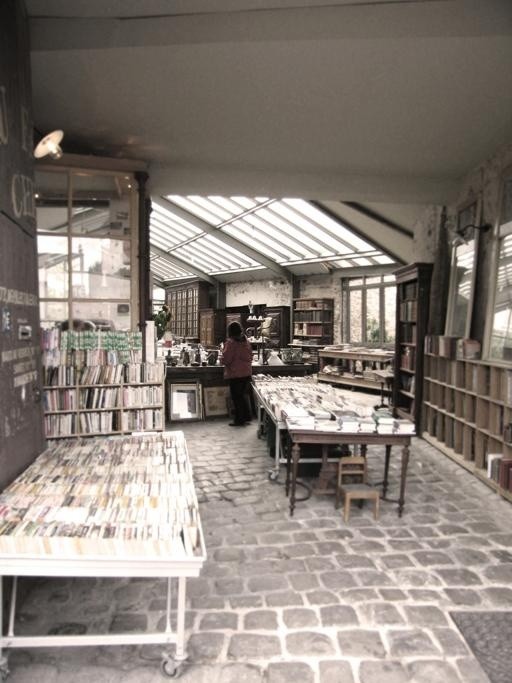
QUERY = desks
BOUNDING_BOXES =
[0,432,204,677]
[315,347,394,397]
[251,368,414,517]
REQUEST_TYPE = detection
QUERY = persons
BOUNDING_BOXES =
[221,321,253,426]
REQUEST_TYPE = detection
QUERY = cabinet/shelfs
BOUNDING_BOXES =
[391,260,432,435]
[196,296,332,371]
[420,349,511,503]
[41,359,164,438]
[163,281,208,345]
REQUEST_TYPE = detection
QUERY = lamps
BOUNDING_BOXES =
[449,223,490,245]
[28,123,63,159]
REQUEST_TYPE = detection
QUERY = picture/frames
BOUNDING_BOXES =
[168,381,231,422]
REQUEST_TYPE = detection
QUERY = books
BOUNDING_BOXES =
[42,322,164,436]
[487,453,512,491]
[504,375,512,444]
[322,343,396,383]
[424,335,482,360]
[395,285,418,416]
[0,431,207,577]
[251,372,414,434]
[294,299,330,364]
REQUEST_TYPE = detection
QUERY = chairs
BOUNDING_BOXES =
[332,454,379,525]
